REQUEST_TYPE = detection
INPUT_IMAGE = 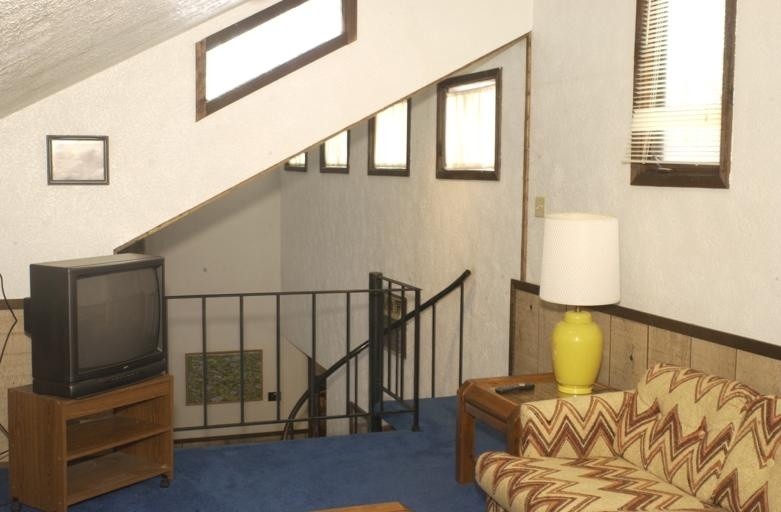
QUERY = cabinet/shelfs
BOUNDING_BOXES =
[7,374,174,512]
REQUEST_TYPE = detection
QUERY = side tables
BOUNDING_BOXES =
[455,372,623,484]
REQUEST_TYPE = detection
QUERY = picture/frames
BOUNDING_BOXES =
[383,290,407,360]
[45,135,110,186]
[184,349,264,407]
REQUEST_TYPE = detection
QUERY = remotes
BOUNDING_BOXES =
[496,382,535,396]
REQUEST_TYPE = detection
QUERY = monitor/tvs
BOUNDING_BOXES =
[24,252,168,398]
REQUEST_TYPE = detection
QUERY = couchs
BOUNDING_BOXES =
[474,362,780,512]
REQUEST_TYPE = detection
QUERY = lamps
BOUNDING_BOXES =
[539,213,621,396]
